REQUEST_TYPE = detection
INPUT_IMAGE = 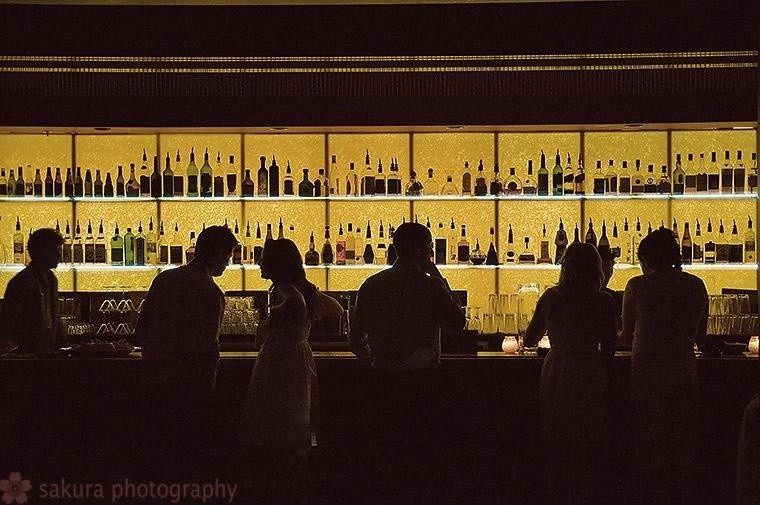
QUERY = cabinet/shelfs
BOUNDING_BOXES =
[336,289,363,337]
[502,336,519,354]
[747,335,760,354]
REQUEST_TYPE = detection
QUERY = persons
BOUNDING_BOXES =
[618,227,728,470]
[348,223,458,386]
[598,244,624,311]
[523,241,618,441]
[132,224,224,393]
[3,228,67,353]
[237,240,342,452]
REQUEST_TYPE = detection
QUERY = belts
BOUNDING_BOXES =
[96,285,147,336]
[463,305,481,333]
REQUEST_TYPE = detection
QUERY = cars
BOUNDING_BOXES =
[54,296,91,338]
[702,292,758,337]
[216,294,259,334]
[482,290,528,336]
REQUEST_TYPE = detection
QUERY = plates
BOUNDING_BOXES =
[1,192,759,271]
